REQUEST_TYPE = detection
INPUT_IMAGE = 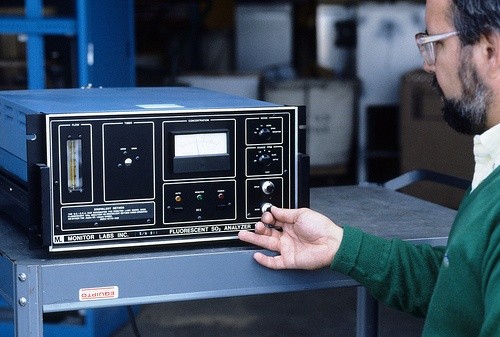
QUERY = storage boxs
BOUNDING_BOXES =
[401,69,476,210]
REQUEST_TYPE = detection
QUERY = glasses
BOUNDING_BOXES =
[415,30,461,65]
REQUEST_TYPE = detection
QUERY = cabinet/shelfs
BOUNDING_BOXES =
[259,77,354,168]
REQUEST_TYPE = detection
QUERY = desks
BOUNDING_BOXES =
[0,184,460,337]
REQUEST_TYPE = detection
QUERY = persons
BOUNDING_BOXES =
[237,0,500,337]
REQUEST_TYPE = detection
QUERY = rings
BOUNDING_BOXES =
[263,219,276,229]
